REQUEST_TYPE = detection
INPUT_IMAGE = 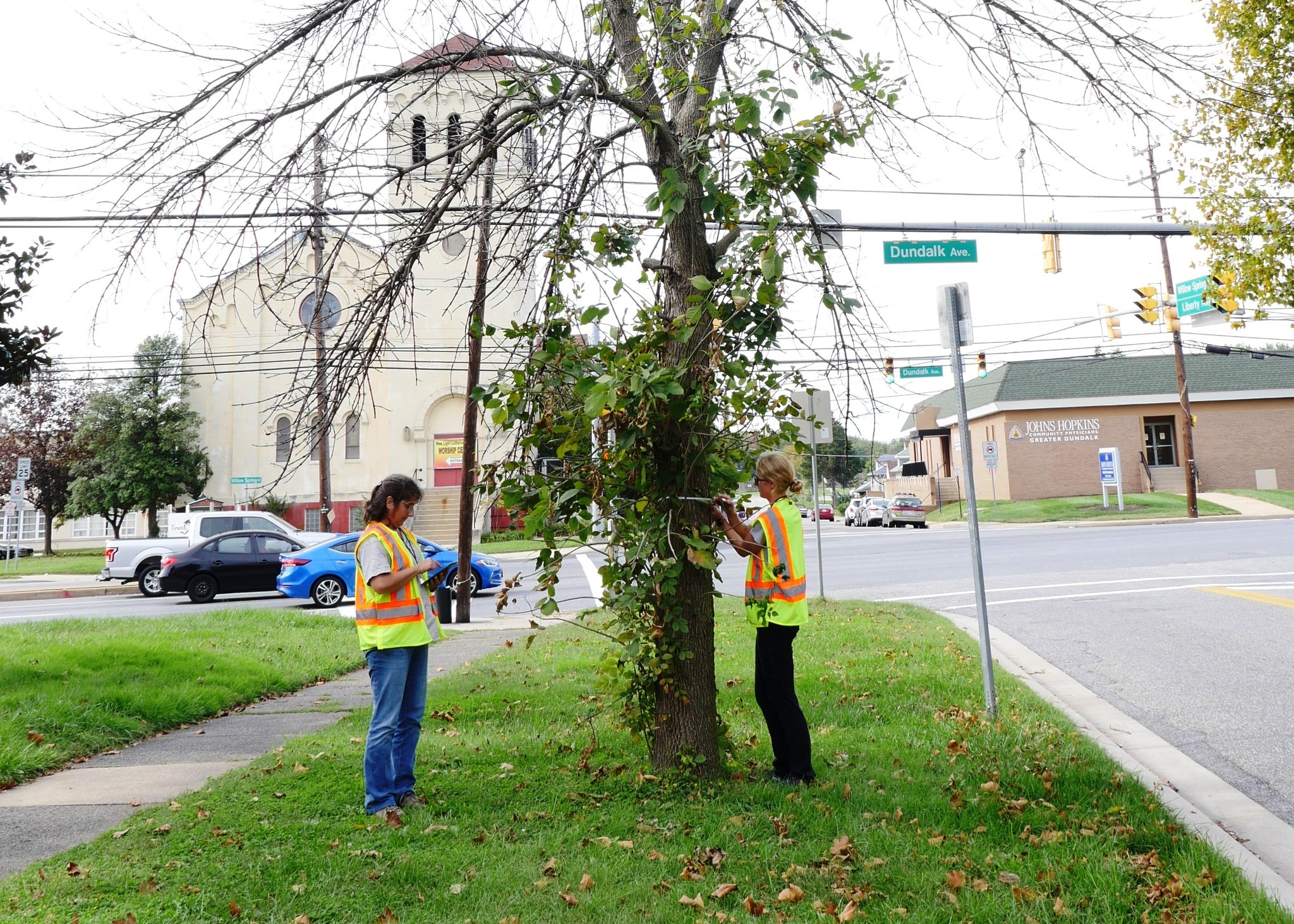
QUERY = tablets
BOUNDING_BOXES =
[420,561,458,586]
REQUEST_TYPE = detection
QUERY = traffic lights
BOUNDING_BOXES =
[1132,285,1160,324]
[1208,270,1238,312]
[1162,294,1181,332]
[977,351,987,378]
[1104,305,1122,338]
[885,356,894,384]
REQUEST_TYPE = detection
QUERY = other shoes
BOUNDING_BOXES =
[763,769,809,785]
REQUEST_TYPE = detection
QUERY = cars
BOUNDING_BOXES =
[0,544,33,560]
[277,531,504,608]
[861,498,891,527]
[732,495,742,505]
[882,493,926,529]
[157,529,311,604]
[811,504,835,522]
[795,504,808,518]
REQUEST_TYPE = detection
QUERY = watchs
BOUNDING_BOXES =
[716,518,727,527]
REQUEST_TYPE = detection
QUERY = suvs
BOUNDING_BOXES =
[855,495,884,527]
[843,498,862,527]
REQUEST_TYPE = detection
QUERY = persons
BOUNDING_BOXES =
[353,473,448,826]
[713,451,815,785]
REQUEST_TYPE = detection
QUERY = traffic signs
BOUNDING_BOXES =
[432,437,478,469]
[5,502,14,516]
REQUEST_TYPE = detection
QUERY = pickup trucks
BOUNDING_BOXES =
[94,510,343,597]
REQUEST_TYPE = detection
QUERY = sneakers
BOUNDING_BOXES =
[401,793,424,811]
[375,804,405,819]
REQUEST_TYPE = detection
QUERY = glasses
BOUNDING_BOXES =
[400,500,414,511]
[754,475,769,485]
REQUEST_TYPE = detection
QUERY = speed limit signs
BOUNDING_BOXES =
[17,458,31,480]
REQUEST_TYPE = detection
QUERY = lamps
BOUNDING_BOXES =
[403,425,411,441]
